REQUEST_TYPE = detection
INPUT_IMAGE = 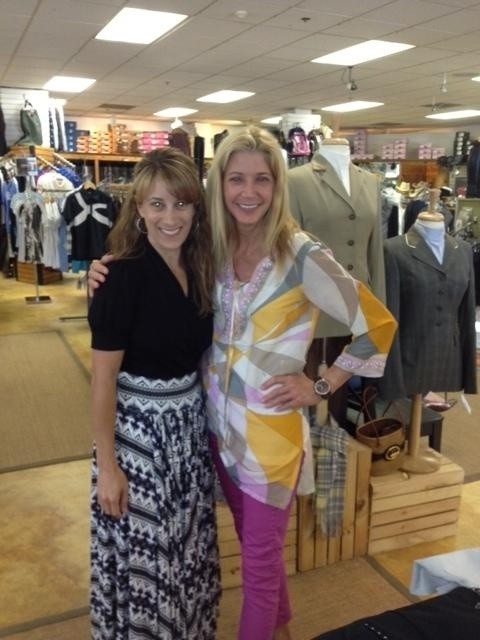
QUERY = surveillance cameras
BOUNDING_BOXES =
[346,79,360,93]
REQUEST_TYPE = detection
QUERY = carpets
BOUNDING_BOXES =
[0,330,93,474]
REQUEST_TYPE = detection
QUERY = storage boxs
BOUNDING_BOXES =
[64,122,170,154]
[349,129,479,161]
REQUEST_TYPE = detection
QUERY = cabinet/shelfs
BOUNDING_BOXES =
[58,148,145,188]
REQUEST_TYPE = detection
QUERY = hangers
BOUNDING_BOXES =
[23,94,33,108]
[24,195,34,203]
[82,173,96,190]
[43,190,54,204]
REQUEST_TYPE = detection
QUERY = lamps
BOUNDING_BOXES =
[341,67,357,91]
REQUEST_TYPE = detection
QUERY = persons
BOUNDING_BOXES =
[87,146,221,640]
[89,124,401,640]
[287,138,386,342]
[384,214,477,400]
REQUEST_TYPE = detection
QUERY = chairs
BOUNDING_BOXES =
[330,337,445,454]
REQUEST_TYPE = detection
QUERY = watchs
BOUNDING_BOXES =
[313,373,333,398]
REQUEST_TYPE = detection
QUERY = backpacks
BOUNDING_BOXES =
[287,126,311,157]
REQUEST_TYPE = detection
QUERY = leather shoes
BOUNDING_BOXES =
[422,398,457,412]
